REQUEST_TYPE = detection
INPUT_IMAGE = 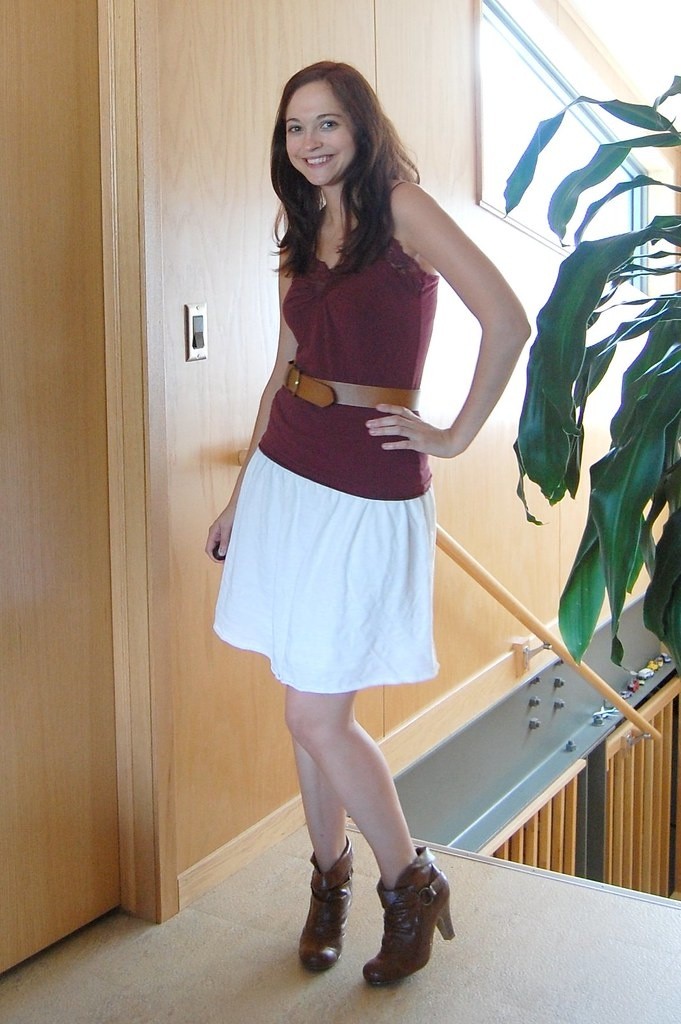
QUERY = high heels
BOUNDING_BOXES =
[299,835,354,972]
[362,846,455,986]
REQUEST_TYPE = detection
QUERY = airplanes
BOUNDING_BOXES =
[591,705,620,721]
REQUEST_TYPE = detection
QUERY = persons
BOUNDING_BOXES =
[205,60,534,987]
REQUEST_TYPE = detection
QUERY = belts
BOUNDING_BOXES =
[283,361,421,412]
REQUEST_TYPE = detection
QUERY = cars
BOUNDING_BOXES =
[619,656,664,700]
[660,653,672,663]
